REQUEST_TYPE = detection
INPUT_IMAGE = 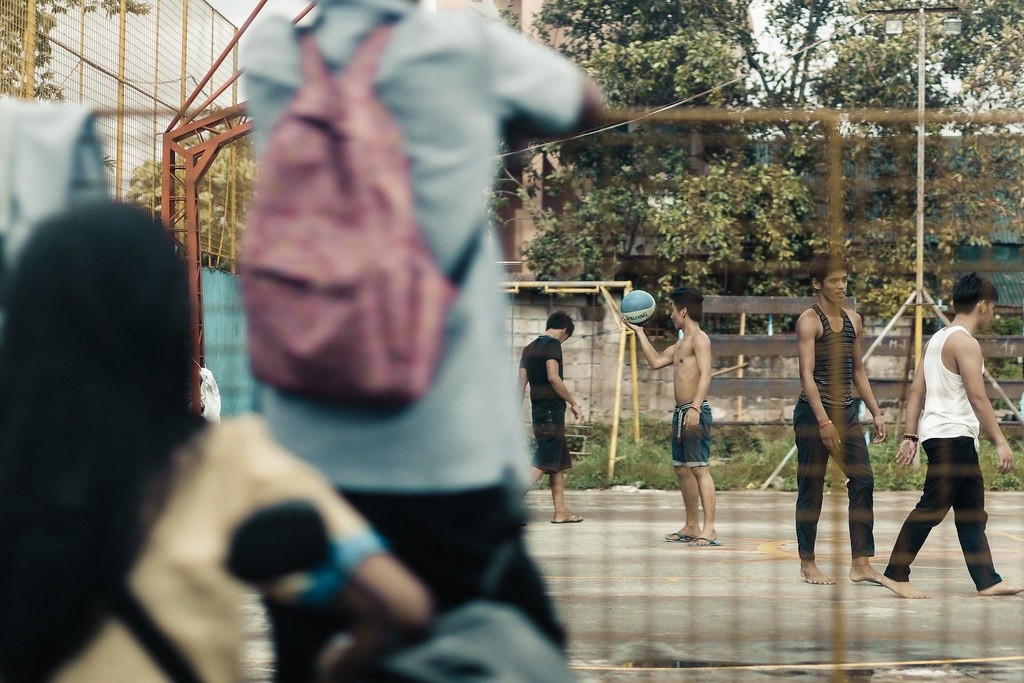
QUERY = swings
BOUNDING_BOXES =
[546,292,597,456]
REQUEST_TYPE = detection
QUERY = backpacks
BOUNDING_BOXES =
[245,15,490,404]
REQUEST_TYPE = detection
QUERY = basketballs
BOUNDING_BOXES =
[620,289,656,327]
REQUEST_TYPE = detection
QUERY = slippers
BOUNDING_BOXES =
[550,515,583,523]
[688,537,723,546]
[665,532,696,542]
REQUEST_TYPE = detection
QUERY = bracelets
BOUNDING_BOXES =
[818,419,832,429]
[902,432,919,444]
[690,404,701,414]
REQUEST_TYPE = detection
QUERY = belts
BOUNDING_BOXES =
[675,401,709,443]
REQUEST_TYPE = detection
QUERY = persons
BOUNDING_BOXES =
[516,309,583,524]
[241,0,614,683]
[0,197,431,683]
[619,287,723,547]
[882,272,1024,599]
[792,249,886,585]
